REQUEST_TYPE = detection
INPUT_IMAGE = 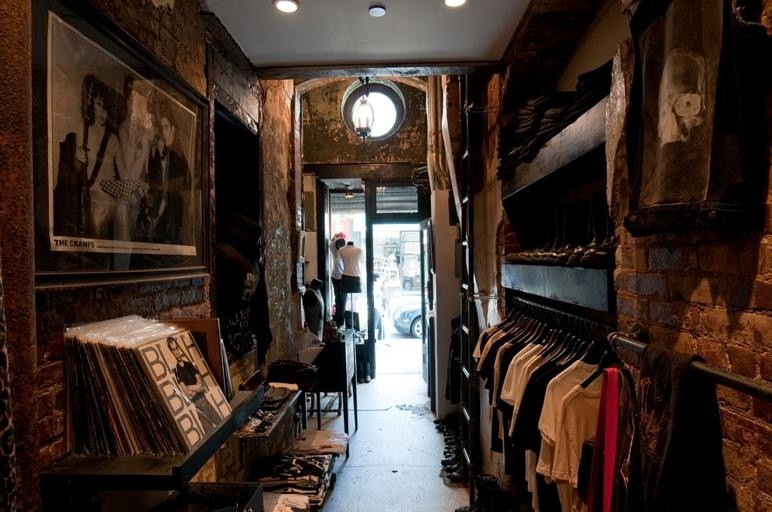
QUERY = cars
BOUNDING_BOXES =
[329,286,387,340]
[401,270,422,290]
[395,302,429,339]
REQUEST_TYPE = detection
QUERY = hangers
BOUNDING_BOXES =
[495,295,628,389]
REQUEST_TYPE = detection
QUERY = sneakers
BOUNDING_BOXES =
[433,419,469,487]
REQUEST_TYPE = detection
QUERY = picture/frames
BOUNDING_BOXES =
[31,1,212,291]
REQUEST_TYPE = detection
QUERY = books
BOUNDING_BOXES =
[247,428,347,511]
[60,314,233,453]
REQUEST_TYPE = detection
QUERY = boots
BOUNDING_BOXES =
[455,473,498,512]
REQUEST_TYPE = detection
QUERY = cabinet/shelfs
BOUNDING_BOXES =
[300,328,358,458]
[40,369,268,512]
[500,93,617,313]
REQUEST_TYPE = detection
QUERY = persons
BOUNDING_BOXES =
[165,337,221,437]
[302,277,325,340]
[328,231,346,329]
[338,242,363,293]
[55,89,192,245]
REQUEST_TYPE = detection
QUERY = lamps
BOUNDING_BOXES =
[349,78,376,141]
